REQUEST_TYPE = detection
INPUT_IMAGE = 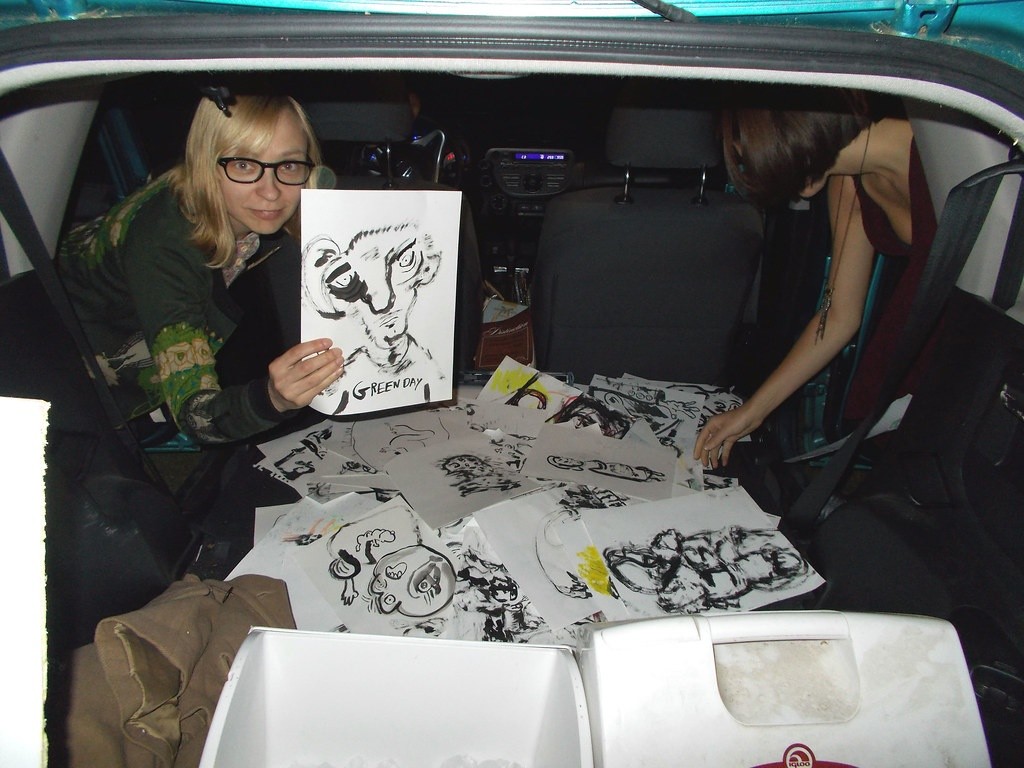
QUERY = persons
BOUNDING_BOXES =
[692,81,947,469]
[53,80,346,498]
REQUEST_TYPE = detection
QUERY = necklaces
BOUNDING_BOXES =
[813,124,871,344]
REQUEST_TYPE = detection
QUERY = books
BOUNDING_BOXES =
[475,297,536,376]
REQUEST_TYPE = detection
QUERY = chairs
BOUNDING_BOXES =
[286,70,485,372]
[528,75,764,387]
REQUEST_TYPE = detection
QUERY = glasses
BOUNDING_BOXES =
[218,157,315,186]
